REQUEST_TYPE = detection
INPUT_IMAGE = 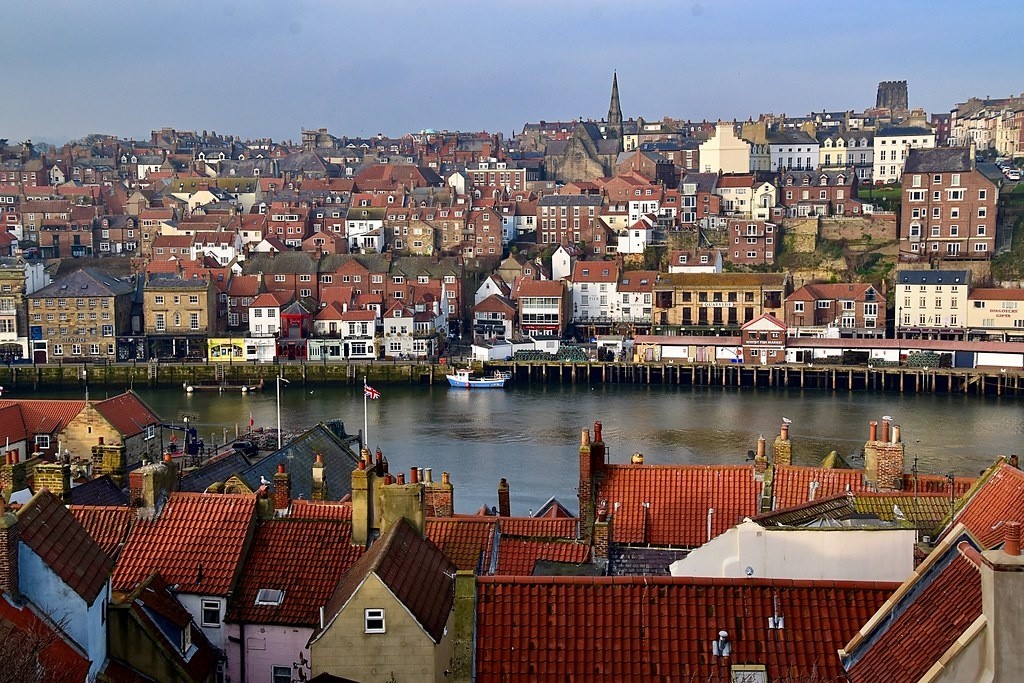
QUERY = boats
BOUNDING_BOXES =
[446,368,512,387]
[182,377,265,392]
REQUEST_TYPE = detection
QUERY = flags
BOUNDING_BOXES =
[364,383,381,400]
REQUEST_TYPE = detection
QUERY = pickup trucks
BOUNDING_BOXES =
[224,441,259,456]
